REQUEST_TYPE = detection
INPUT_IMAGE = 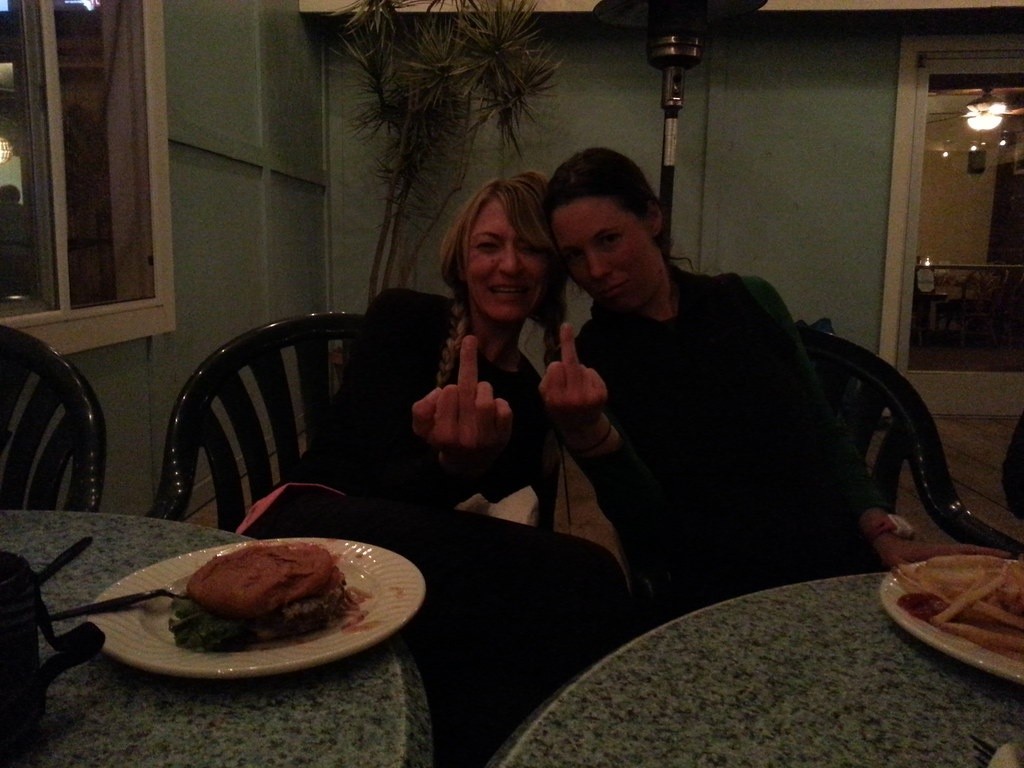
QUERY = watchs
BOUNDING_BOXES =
[867,514,915,548]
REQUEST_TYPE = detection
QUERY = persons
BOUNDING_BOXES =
[539,146,1011,634]
[280,170,631,726]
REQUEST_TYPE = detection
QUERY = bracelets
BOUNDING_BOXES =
[569,424,612,453]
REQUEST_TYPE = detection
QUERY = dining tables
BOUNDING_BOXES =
[0,509,434,766]
[482,572,1024,768]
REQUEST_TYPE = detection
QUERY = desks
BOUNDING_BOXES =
[915,264,1023,348]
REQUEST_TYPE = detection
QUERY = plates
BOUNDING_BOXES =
[881,555,1024,685]
[86,536,425,678]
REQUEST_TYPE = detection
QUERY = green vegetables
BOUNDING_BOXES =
[168,598,258,650]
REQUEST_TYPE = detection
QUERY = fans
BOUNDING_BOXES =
[927,88,1024,125]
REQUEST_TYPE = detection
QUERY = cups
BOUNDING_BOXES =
[0,551,106,765]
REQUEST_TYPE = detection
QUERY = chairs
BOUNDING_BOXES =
[0,313,1024,561]
[910,259,1023,350]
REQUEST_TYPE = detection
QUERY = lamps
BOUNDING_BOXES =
[966,112,1003,132]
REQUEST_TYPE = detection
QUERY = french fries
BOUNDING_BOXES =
[890,554,1024,656]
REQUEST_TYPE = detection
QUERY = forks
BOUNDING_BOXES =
[970,735,996,768]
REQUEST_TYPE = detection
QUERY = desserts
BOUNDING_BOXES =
[187,542,343,639]
